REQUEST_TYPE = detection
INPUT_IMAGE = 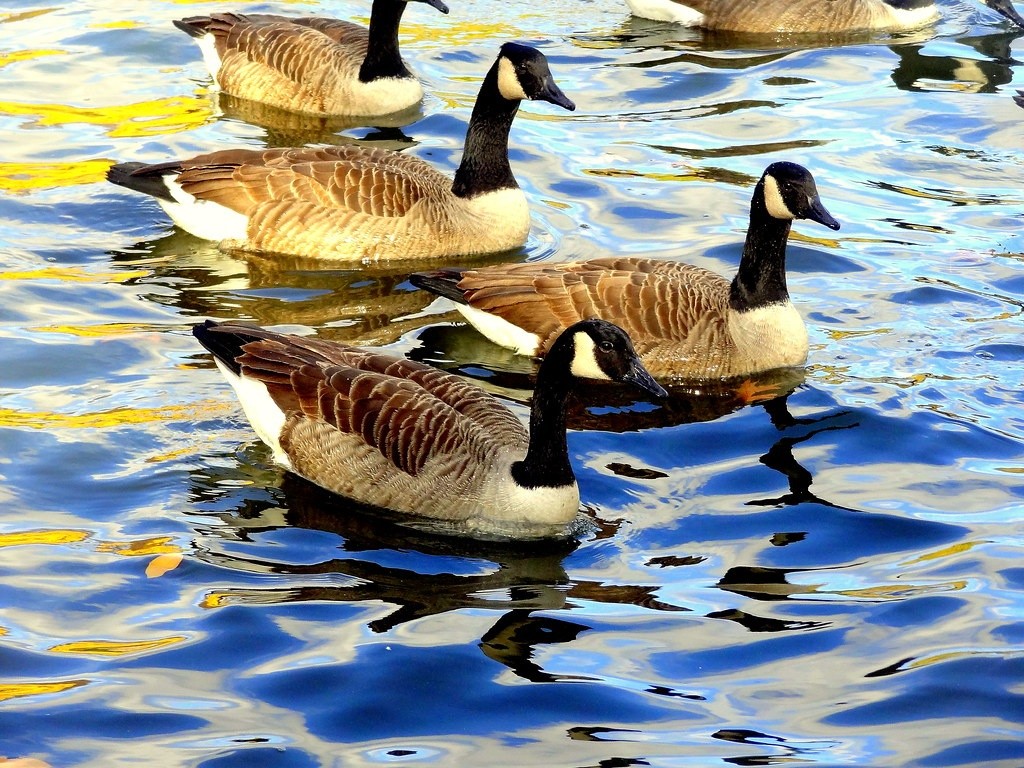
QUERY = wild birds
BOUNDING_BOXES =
[192,317,670,540]
[407,161,842,379]
[104,41,575,263]
[623,0,944,37]
[173,1,450,119]
